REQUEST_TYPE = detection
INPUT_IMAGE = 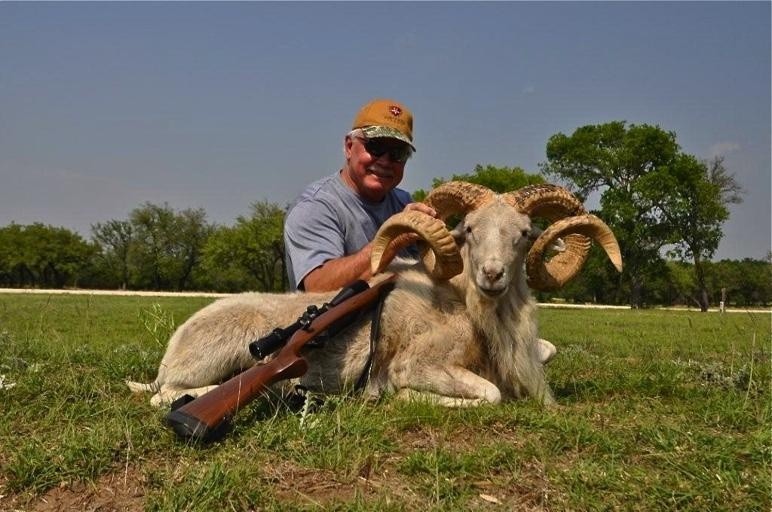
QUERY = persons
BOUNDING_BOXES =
[284,101,436,294]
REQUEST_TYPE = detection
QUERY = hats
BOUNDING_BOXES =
[351,97,418,152]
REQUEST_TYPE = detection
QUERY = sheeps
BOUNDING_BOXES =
[125,179,622,415]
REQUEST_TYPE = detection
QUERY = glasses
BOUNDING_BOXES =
[358,137,410,163]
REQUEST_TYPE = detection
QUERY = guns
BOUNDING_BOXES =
[168,262,399,448]
